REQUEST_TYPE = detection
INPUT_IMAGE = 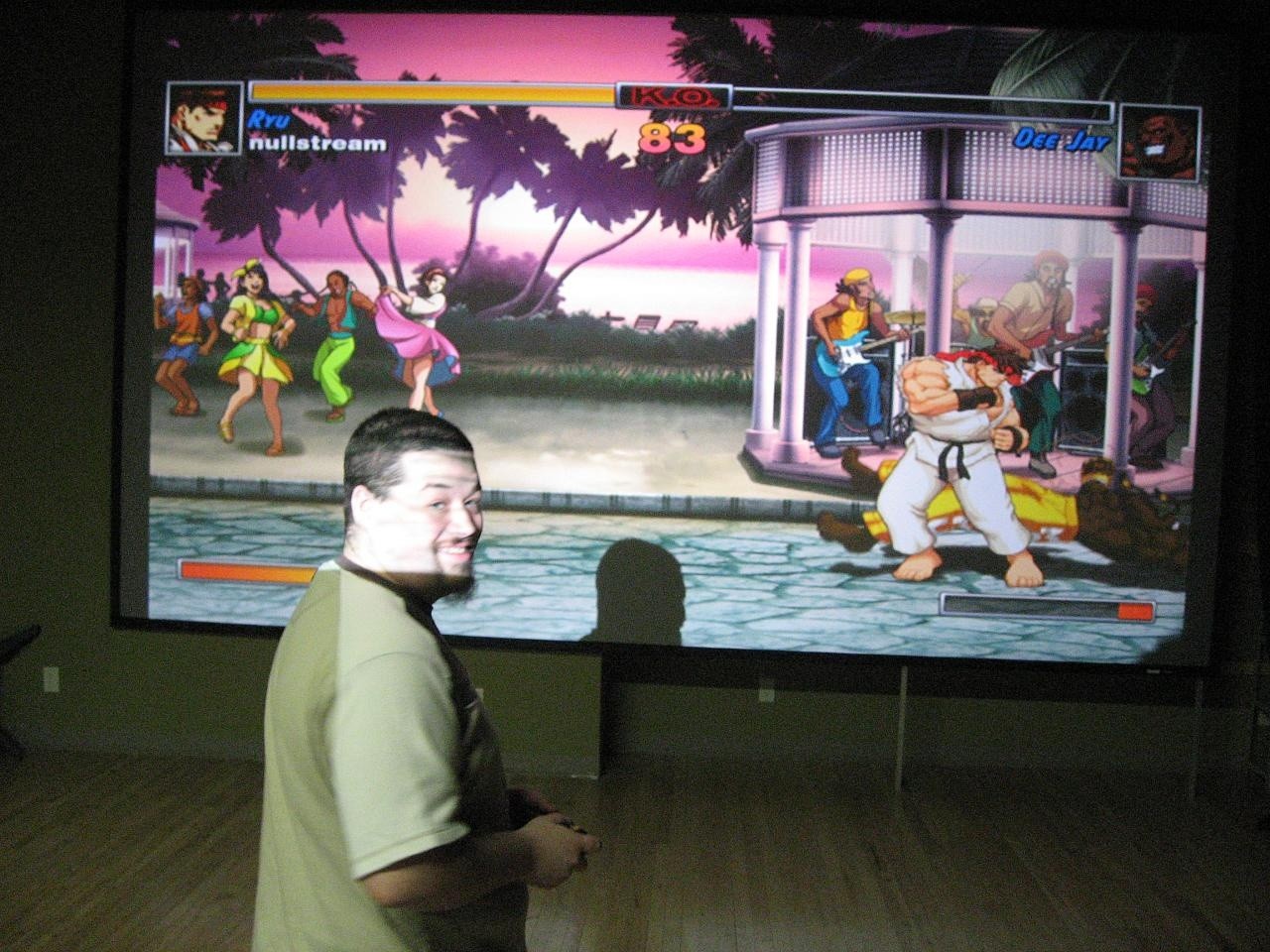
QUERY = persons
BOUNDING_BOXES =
[250,408,600,952]
